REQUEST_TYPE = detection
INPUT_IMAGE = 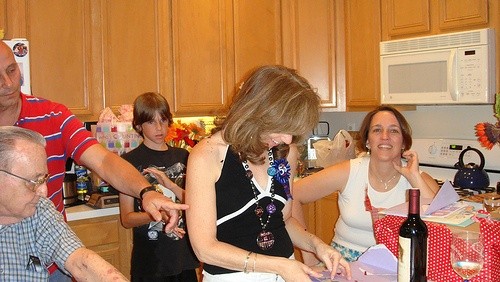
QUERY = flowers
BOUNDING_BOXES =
[164,118,216,153]
[474,93,500,150]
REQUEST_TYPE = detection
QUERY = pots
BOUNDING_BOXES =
[62,174,78,205]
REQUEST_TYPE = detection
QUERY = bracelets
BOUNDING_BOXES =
[253,253,257,272]
[244,251,253,273]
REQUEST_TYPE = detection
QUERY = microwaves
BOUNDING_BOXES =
[380,26,497,105]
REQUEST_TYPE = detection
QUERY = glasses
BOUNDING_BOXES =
[0,168,49,191]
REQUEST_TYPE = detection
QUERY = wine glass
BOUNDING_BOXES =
[450,231,483,282]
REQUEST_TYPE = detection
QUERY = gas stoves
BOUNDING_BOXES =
[399,138,498,198]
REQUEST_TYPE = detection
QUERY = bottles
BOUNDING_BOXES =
[74,163,88,195]
[396,190,428,282]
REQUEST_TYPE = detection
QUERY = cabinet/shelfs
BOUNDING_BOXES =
[67,192,340,282]
[0,0,500,125]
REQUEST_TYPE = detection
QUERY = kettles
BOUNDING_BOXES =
[453,146,490,189]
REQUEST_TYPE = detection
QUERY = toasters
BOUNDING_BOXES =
[307,135,332,168]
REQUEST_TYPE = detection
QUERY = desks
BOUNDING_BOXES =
[373,192,500,282]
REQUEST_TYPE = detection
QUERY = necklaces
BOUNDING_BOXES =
[244,149,276,250]
[372,169,398,189]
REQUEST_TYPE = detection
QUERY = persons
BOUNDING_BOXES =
[118,91,202,282]
[290,104,440,268]
[184,65,351,282]
[0,40,191,282]
[0,126,134,282]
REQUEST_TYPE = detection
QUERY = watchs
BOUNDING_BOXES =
[140,184,163,200]
